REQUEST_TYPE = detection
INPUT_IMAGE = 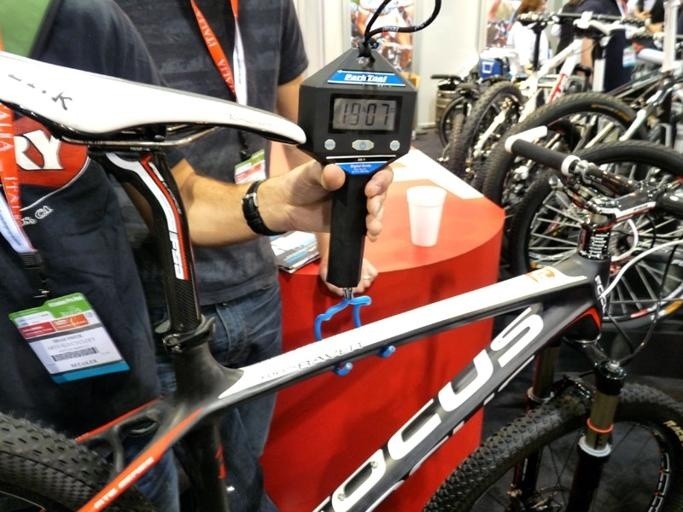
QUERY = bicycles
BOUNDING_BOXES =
[0,51,683,512]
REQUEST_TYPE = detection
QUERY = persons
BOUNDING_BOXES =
[0,0,397,511]
[504,1,683,116]
[104,0,383,511]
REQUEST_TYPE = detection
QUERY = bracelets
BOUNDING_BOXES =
[241,179,288,236]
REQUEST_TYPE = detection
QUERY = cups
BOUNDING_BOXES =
[405,186,446,248]
